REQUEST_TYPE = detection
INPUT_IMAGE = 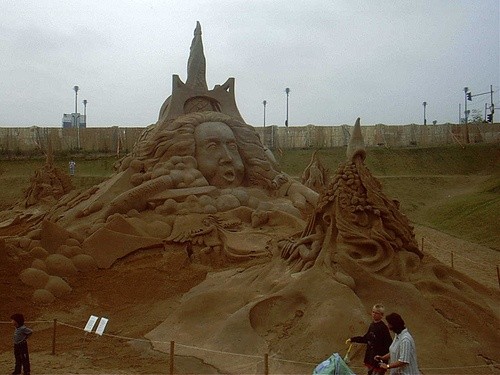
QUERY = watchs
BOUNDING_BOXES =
[386,364,390,368]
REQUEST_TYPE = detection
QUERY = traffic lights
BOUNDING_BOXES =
[487,113,492,122]
[467,92,472,100]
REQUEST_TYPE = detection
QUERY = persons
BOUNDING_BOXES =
[9,314,34,375]
[137,112,244,195]
[374,313,419,375]
[69,159,76,176]
[347,304,393,375]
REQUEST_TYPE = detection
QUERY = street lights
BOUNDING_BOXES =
[82,99,87,128]
[423,101,426,126]
[74,85,79,113]
[285,88,290,127]
[463,87,468,124]
[263,100,267,128]
